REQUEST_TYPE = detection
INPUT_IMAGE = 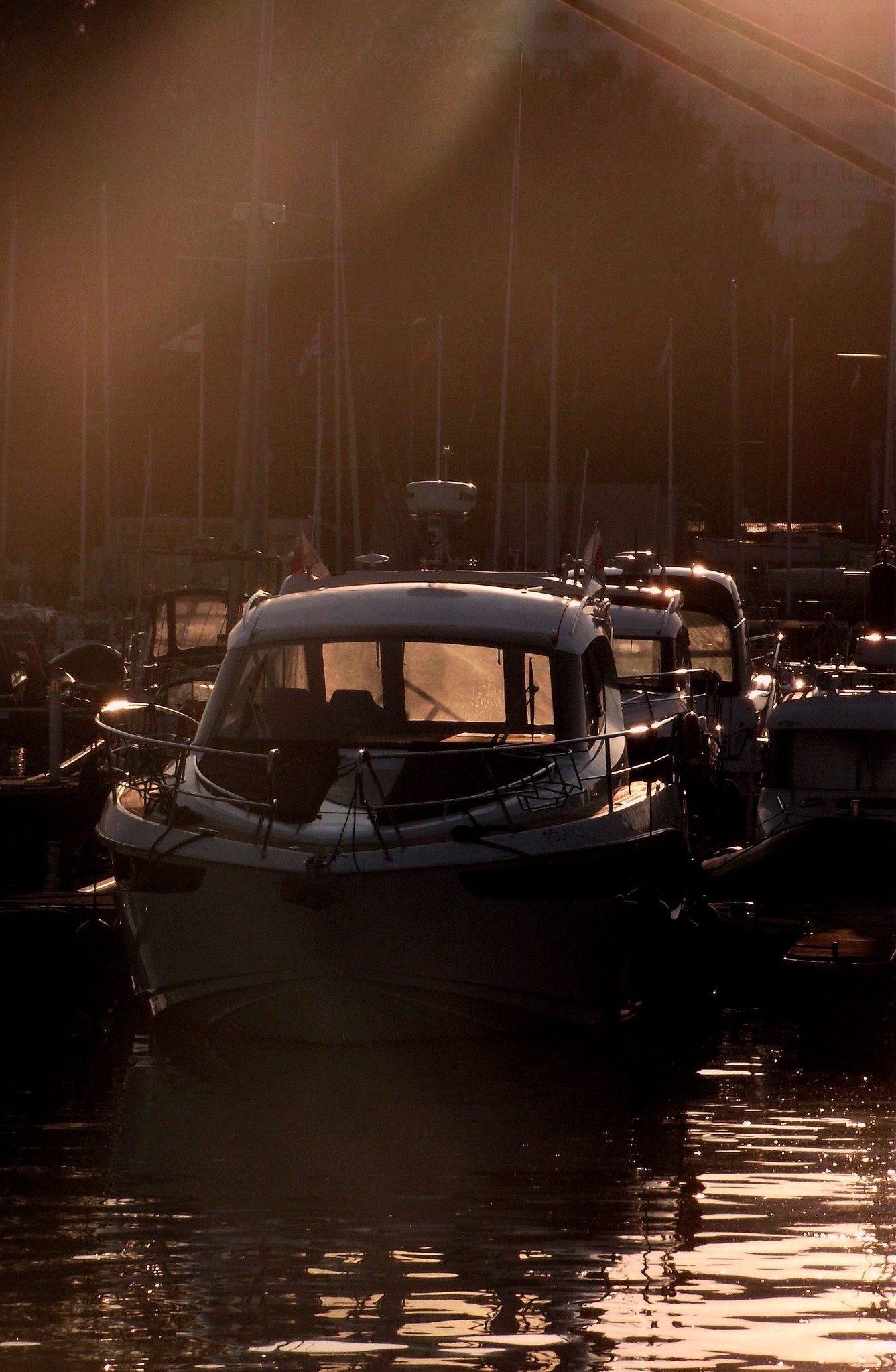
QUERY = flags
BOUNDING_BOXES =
[291,527,329,580]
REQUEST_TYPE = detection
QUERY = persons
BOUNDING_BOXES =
[812,612,838,664]
[838,619,859,662]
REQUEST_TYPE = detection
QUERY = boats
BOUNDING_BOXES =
[1,40,896,1066]
